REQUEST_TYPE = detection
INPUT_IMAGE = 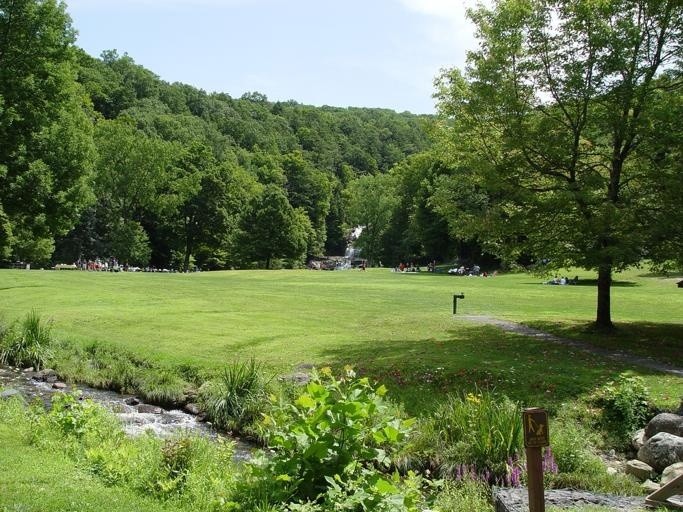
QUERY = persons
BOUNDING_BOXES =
[553,275,568,286]
[458,265,497,277]
